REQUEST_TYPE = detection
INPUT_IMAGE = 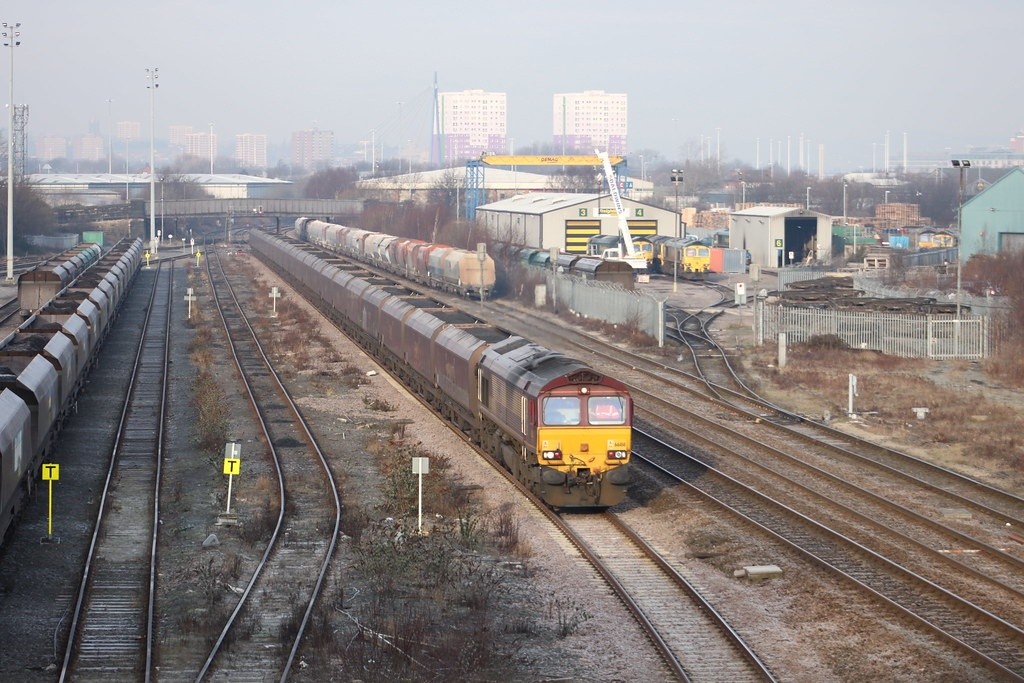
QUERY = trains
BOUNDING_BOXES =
[0,236,143,546]
[489,239,634,290]
[249,228,634,515]
[587,234,711,281]
[294,217,496,301]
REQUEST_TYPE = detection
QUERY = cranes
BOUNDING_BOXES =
[465,154,627,224]
[11,103,29,186]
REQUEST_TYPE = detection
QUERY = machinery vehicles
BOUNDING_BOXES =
[594,148,650,283]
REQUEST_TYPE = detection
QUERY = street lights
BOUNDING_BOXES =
[2,22,20,283]
[853,224,859,254]
[371,128,375,174]
[885,191,891,204]
[209,121,216,174]
[671,168,684,293]
[741,182,745,210]
[843,184,848,223]
[807,187,811,210]
[145,67,159,254]
[951,159,971,316]
[915,191,922,225]
[105,99,113,174]
